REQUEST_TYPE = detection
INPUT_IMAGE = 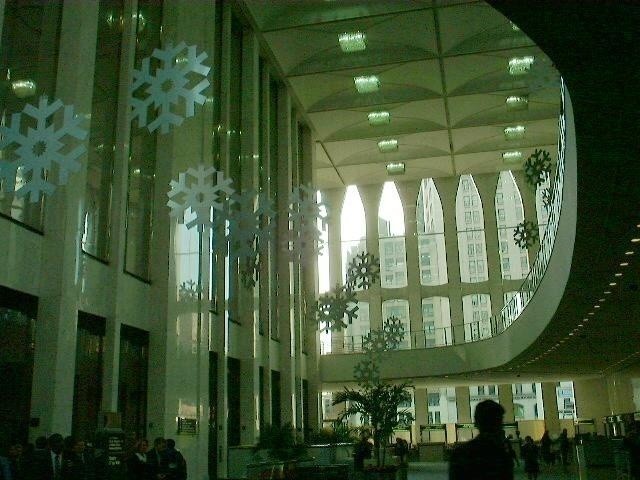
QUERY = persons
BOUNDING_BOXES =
[620,430,640,480]
[555,427,572,467]
[520,436,541,480]
[391,436,405,463]
[540,429,555,474]
[402,439,410,468]
[0,433,190,480]
[449,397,515,480]
[502,438,520,468]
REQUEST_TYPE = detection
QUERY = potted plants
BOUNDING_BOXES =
[334,379,413,478]
